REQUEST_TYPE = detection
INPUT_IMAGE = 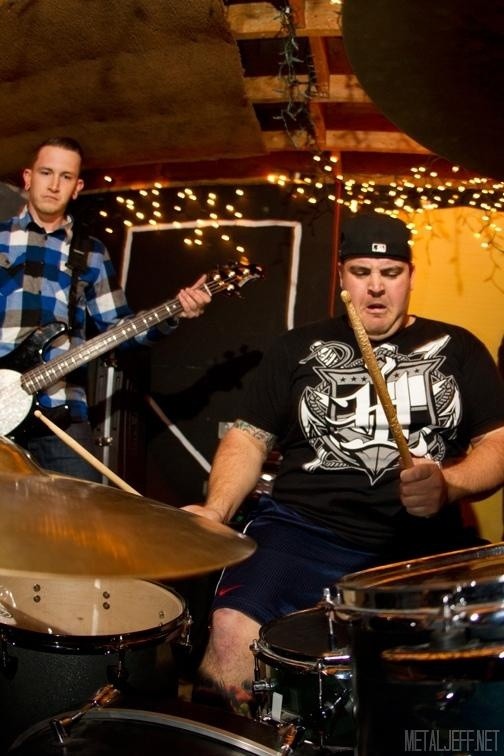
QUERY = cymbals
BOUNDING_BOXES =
[0,433,256,577]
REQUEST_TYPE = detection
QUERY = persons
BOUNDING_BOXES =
[180,212,504,701]
[1,137,213,484]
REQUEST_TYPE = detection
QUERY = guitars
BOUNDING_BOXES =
[0,264,264,439]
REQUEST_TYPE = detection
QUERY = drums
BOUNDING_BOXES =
[333,544,504,755]
[0,566,196,721]
[254,609,356,756]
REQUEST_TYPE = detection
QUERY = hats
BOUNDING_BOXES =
[341,210,412,265]
